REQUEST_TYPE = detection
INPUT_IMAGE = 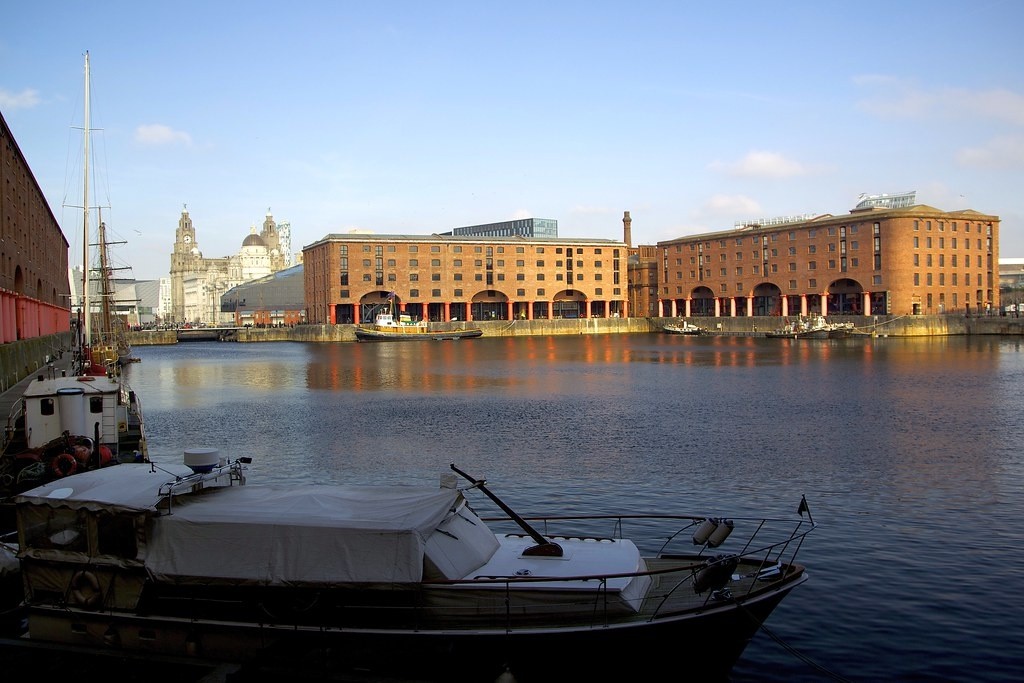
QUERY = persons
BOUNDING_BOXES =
[58,347,63,359]
[244,319,310,327]
[129,321,180,331]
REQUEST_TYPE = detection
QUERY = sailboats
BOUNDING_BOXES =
[0,47,150,531]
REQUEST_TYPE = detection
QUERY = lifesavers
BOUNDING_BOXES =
[70,571,100,605]
[51,453,78,478]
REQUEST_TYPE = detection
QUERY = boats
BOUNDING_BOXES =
[662,314,710,336]
[764,310,885,340]
[0,435,819,683]
[353,292,483,343]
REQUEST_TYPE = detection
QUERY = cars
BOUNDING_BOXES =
[170,321,233,330]
